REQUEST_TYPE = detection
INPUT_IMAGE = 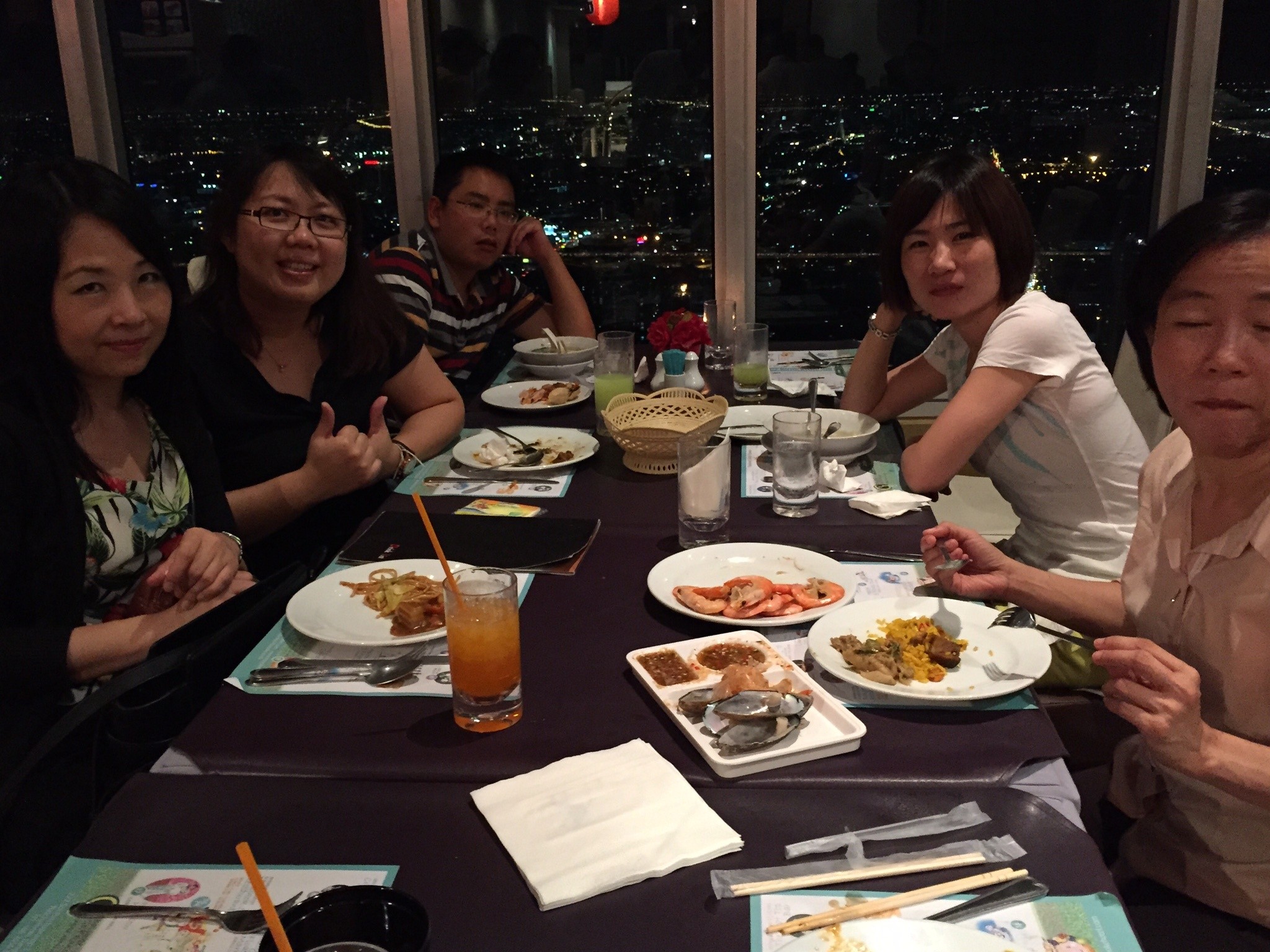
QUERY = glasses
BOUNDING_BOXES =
[444,199,519,226]
[236,206,352,238]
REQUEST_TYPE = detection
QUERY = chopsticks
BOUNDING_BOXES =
[731,852,1029,934]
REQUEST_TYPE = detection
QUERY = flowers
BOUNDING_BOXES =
[647,308,712,356]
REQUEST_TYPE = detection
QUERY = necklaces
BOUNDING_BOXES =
[257,317,315,377]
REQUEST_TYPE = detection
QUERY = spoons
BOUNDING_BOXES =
[246,659,424,686]
[809,378,818,413]
[469,452,544,472]
[802,358,853,368]
[821,422,841,439]
[933,539,972,570]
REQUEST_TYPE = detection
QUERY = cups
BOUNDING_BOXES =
[664,370,686,396]
[772,410,822,518]
[596,331,636,438]
[257,885,428,952]
[442,566,524,731]
[702,298,736,370]
[732,321,770,402]
[677,435,729,549]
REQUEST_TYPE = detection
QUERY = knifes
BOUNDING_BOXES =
[424,477,560,485]
[797,544,925,564]
[277,656,451,665]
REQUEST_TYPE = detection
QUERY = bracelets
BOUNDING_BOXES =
[221,530,243,568]
[867,314,900,339]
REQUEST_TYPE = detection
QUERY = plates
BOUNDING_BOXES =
[453,426,600,471]
[809,596,1052,700]
[622,630,867,778]
[715,405,799,441]
[287,558,487,646]
[647,542,857,627]
[480,380,592,408]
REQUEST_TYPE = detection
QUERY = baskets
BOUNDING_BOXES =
[599,387,728,475]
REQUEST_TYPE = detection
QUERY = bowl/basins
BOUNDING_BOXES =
[763,407,883,451]
[759,431,877,467]
[513,352,595,377]
[514,335,600,362]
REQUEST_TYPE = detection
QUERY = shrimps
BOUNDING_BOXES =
[521,383,553,404]
[668,574,845,617]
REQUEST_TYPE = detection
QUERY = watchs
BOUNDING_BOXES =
[390,439,418,482]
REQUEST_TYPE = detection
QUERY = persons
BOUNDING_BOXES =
[0,149,261,771]
[159,135,465,599]
[921,178,1270,951]
[359,147,598,445]
[839,149,1152,697]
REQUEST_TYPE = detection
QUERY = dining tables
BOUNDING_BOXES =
[0,374,1148,950]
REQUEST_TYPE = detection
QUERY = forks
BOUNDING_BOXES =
[69,892,304,933]
[988,608,1097,654]
[809,352,856,361]
[248,637,432,679]
[484,423,551,452]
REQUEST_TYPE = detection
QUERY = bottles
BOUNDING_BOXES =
[685,351,704,392]
[650,353,668,390]
